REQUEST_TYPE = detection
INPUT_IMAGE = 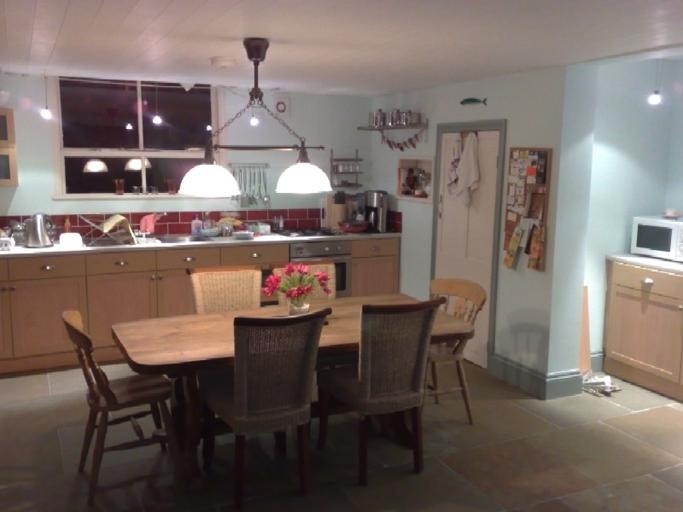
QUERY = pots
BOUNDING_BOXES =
[368,108,420,127]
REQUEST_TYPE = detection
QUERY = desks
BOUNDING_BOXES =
[112,292,475,505]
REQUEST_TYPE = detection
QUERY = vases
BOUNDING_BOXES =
[286,295,310,316]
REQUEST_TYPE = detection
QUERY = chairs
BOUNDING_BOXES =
[420,278,488,426]
[315,298,447,485]
[189,269,263,312]
[62,308,199,508]
[205,305,333,508]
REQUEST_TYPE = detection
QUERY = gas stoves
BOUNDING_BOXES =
[275,228,335,237]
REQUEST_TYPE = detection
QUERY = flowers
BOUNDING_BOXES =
[261,264,333,296]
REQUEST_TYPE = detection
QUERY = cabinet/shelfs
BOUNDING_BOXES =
[221,243,291,306]
[1,252,84,376]
[350,237,398,295]
[331,149,363,189]
[603,261,683,404]
[86,246,157,366]
[156,246,221,316]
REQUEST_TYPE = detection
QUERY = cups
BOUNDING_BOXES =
[0,237,16,251]
[130,186,140,195]
[167,178,176,195]
[151,186,159,195]
[115,179,124,195]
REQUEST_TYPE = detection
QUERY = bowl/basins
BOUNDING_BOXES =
[232,231,254,239]
[337,221,370,232]
[202,228,221,236]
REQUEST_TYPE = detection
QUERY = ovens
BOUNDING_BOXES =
[291,241,351,297]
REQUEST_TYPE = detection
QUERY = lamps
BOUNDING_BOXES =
[40,79,53,120]
[81,108,152,174]
[177,38,333,197]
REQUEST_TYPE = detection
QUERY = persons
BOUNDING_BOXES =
[401,167,427,197]
